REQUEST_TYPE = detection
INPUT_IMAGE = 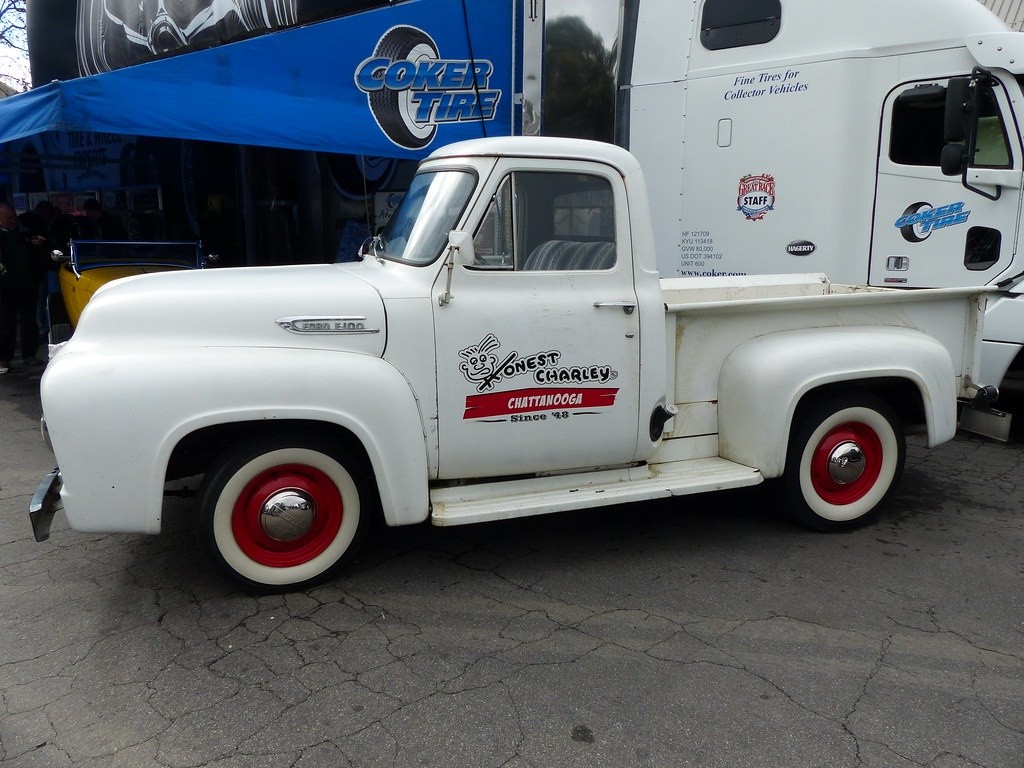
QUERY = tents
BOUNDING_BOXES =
[0,0,546,263]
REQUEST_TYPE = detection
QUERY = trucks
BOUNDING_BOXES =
[23,2,1024,446]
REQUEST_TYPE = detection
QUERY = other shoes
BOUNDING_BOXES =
[26,357,40,365]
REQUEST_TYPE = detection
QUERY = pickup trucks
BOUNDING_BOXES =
[25,132,997,596]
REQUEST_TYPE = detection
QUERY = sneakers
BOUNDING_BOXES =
[0,366,8,374]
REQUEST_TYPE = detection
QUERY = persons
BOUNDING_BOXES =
[17,179,246,327]
[0,198,46,376]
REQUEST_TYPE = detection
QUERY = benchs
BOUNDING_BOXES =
[523,240,617,271]
